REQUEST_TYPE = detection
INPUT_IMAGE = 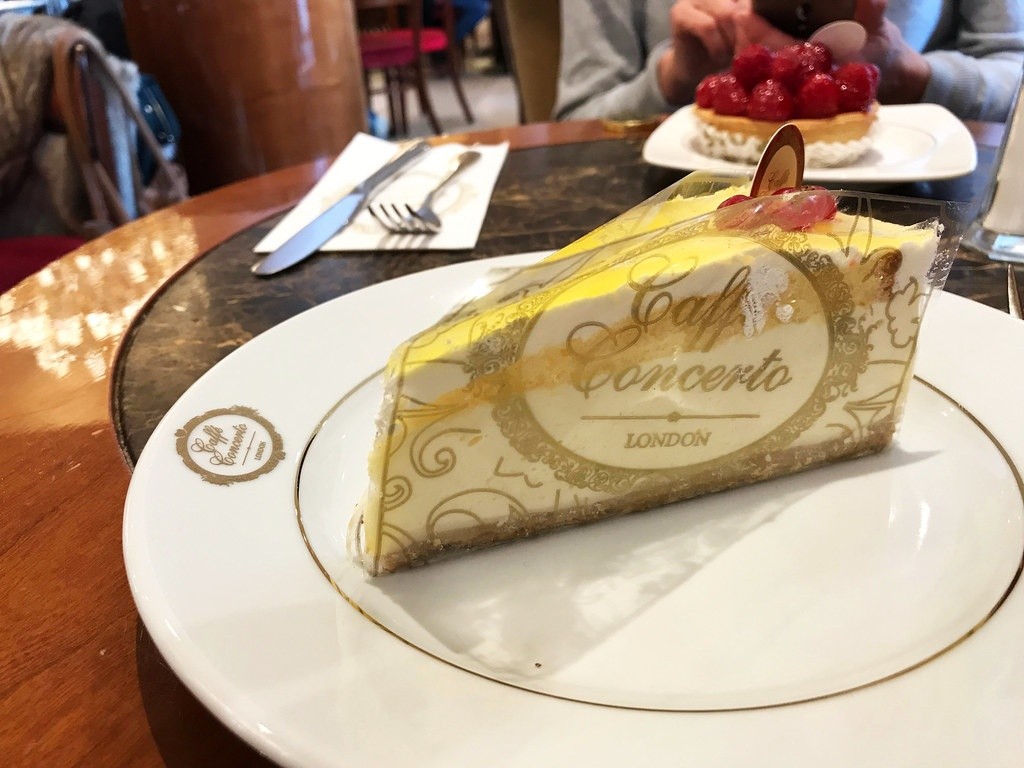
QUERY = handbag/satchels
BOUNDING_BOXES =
[53,25,190,235]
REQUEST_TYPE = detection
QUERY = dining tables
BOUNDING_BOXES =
[0,115,1024,768]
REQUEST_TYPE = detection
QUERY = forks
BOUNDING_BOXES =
[367,151,481,234]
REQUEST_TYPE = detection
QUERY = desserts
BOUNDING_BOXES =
[362,171,972,578]
[693,43,881,168]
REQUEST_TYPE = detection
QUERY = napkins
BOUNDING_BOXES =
[252,132,511,254]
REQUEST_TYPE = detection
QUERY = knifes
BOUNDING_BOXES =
[250,142,433,274]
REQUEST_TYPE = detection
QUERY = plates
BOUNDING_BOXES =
[640,103,978,182]
[120,258,1024,768]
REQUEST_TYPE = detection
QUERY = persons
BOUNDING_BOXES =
[552,2,1024,127]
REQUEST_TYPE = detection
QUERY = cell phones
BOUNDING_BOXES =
[750,0,856,42]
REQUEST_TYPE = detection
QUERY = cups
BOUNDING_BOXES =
[958,62,1024,264]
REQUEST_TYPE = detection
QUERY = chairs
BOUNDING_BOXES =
[361,0,476,136]
